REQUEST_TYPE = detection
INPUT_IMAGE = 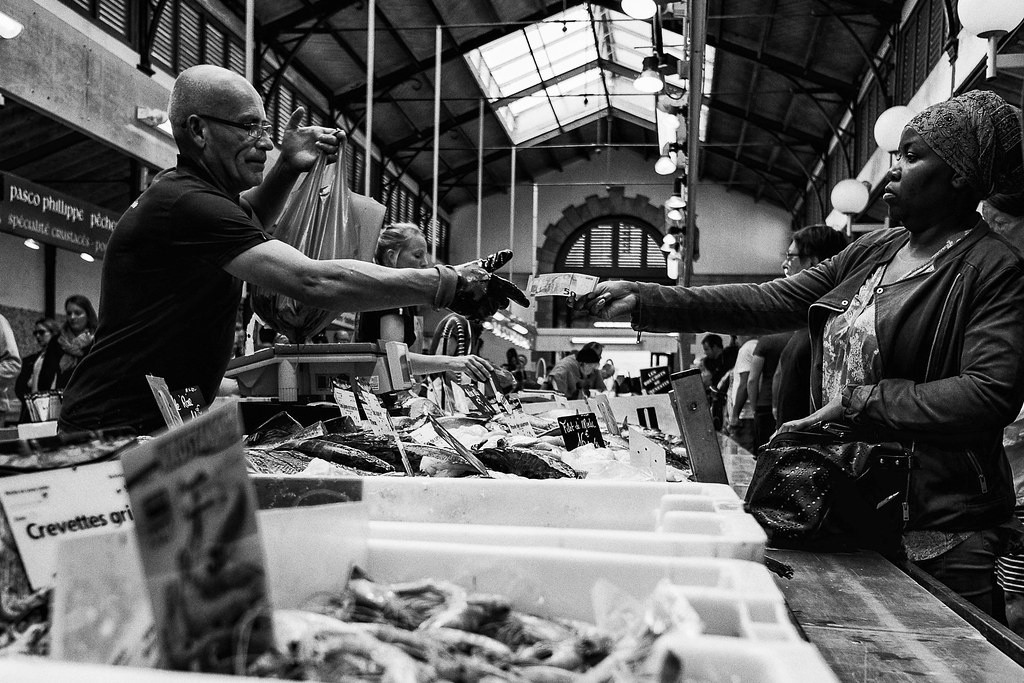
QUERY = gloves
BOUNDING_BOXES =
[433,249,530,325]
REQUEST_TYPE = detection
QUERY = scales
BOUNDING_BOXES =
[223,343,388,402]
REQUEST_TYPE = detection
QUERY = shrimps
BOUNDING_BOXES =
[267,567,652,683]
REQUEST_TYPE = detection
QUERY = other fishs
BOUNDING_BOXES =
[280,410,687,481]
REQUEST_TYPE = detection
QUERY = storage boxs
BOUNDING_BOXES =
[347,473,767,564]
[0,532,840,683]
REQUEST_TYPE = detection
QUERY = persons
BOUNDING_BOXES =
[981,189,1024,639]
[565,91,1024,625]
[350,220,851,458]
[0,294,99,428]
[56,65,531,433]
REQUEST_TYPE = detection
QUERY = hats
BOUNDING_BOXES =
[577,348,600,363]
[904,89,1024,200]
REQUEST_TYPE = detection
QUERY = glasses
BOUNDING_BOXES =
[182,115,273,139]
[785,250,813,263]
[33,329,50,336]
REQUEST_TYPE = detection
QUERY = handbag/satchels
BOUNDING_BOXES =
[745,419,919,549]
[251,128,387,344]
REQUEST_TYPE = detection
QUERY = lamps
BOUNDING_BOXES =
[830,179,892,237]
[136,105,173,139]
[654,149,677,175]
[633,67,664,94]
[665,195,687,208]
[873,106,917,158]
[957,0,1024,82]
